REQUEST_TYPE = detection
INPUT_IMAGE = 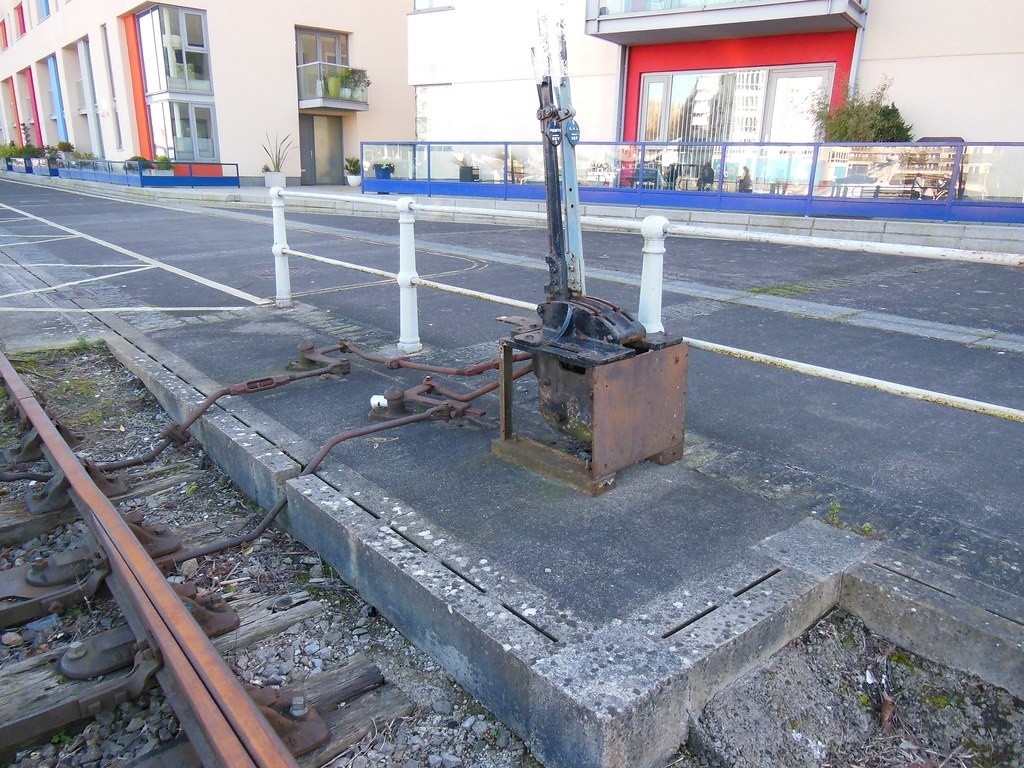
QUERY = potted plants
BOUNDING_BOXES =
[123,156,176,189]
[261,131,304,188]
[327,66,372,102]
[343,156,362,187]
[57,142,75,164]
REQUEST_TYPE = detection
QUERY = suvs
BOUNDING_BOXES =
[613,167,666,190]
[664,162,705,191]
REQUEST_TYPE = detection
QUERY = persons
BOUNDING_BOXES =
[937,172,952,199]
[662,164,681,191]
[910,172,925,200]
[697,162,714,192]
[739,166,752,188]
